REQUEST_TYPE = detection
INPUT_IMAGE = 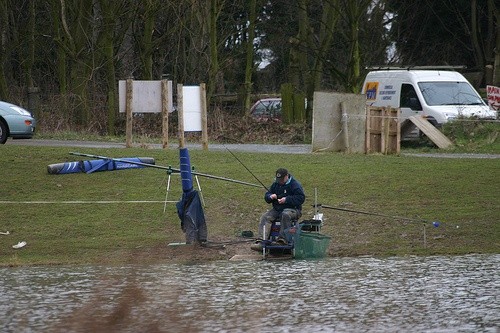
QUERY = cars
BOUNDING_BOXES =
[244,97,282,127]
[0,101,36,145]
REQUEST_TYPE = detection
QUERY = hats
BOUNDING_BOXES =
[276,168,288,181]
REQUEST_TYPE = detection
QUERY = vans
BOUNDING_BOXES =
[361,64,495,144]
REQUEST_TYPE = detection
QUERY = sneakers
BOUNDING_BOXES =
[277,236,285,243]
[251,242,263,253]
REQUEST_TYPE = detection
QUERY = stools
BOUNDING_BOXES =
[261,217,297,261]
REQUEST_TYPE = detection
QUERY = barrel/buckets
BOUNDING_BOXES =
[293,229,332,260]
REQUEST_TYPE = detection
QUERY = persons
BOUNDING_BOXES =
[251,168,305,253]
